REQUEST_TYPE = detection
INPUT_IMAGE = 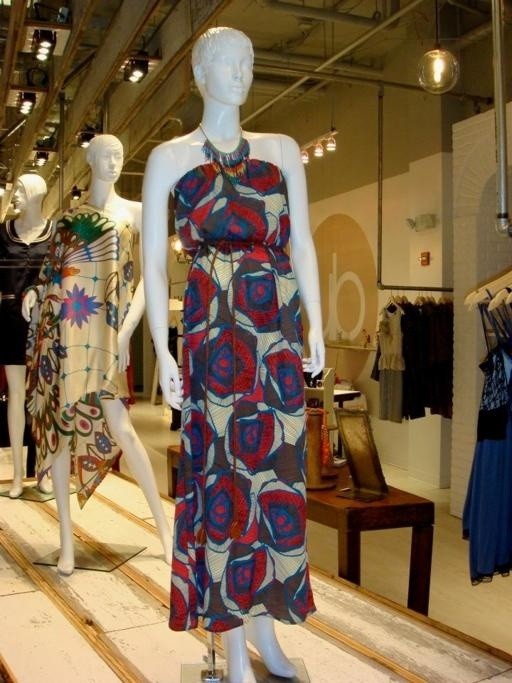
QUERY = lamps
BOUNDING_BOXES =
[16,93,37,116]
[78,134,92,149]
[301,150,309,164]
[171,239,193,263]
[415,2,461,94]
[35,152,48,168]
[72,192,80,200]
[121,61,148,85]
[314,144,324,157]
[31,31,56,61]
[326,137,337,151]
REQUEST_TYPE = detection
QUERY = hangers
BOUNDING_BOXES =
[464,270,512,311]
[379,286,454,315]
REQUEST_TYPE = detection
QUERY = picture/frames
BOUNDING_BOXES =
[334,408,389,492]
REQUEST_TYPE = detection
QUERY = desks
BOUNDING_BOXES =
[333,389,361,458]
[168,444,435,617]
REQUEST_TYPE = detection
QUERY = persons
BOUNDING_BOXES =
[20,134,174,576]
[0,173,53,499]
[142,26,326,683]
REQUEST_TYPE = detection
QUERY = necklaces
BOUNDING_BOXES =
[198,122,251,167]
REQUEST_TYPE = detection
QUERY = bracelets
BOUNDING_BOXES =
[23,285,39,302]
